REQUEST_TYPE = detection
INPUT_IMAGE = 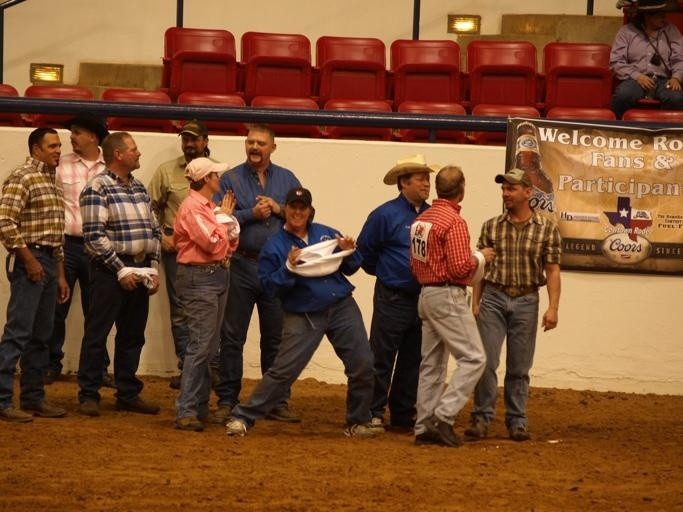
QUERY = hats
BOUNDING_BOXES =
[495,168,533,187]
[184,157,228,183]
[63,109,109,146]
[384,155,441,185]
[178,118,208,138]
[286,188,312,204]
[627,0,678,13]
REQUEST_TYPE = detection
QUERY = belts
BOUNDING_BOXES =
[423,282,466,290]
[65,234,84,246]
[487,282,538,297]
[116,253,152,266]
[28,243,54,256]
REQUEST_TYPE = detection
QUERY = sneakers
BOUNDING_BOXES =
[344,418,530,448]
[1,373,300,437]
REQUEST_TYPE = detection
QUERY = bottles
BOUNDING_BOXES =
[515,121,556,214]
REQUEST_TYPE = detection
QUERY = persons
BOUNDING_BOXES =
[79,133,161,416]
[0,127,65,423]
[356,153,435,434]
[227,188,377,441]
[43,113,117,388]
[465,169,561,440]
[212,125,304,421]
[610,1,683,114]
[148,121,228,391]
[170,157,242,430]
[411,166,496,448]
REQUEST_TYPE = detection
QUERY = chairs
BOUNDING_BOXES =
[315,36,385,101]
[541,41,614,110]
[388,40,463,109]
[241,33,314,96]
[161,27,240,95]
[632,97,676,103]
[466,41,540,107]
